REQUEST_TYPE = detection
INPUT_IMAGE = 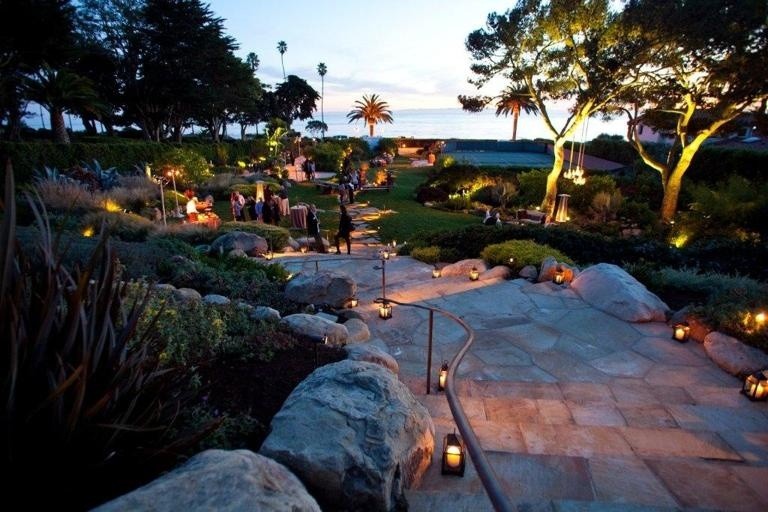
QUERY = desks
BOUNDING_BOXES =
[291,206,307,229]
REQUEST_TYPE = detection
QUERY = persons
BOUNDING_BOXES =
[428,150,436,163]
[184,151,393,255]
[482,209,501,226]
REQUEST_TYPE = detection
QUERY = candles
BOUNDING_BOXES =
[434,272,439,277]
[676,330,685,339]
[380,308,388,317]
[440,374,447,387]
[471,274,477,279]
[750,383,764,397]
[385,252,388,258]
[352,300,357,306]
[556,276,562,283]
[446,448,460,467]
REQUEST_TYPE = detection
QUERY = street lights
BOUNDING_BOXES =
[167,168,187,219]
